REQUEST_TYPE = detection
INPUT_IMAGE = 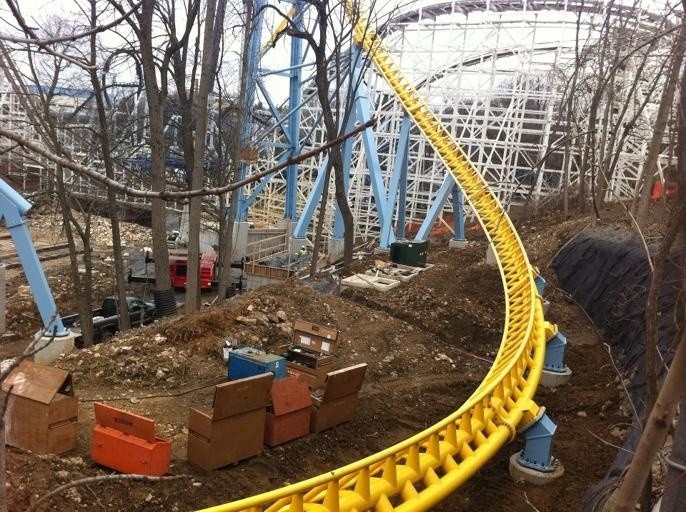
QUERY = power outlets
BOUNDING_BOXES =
[58,294,156,350]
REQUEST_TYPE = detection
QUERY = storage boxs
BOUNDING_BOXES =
[271,319,340,391]
[187,371,274,471]
[91,402,171,477]
[265,375,311,447]
[228,345,286,382]
[0,360,79,460]
[310,362,368,434]
